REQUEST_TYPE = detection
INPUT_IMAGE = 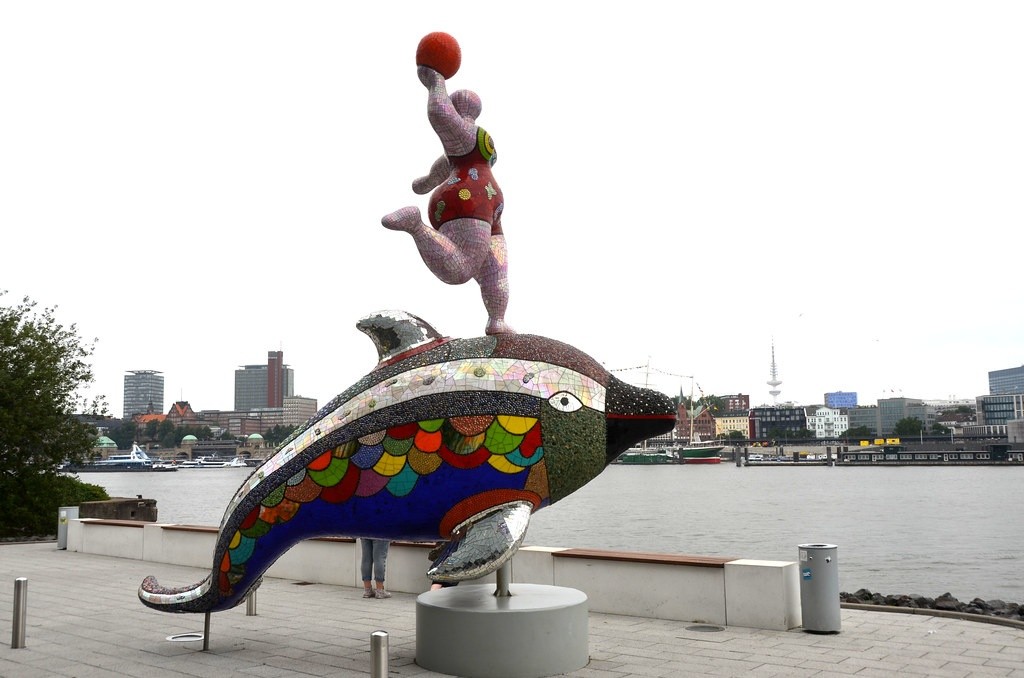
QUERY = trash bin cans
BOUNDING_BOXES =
[798,543,841,633]
[57,507,79,548]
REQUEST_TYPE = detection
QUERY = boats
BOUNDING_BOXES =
[610,445,725,464]
[55,441,179,472]
[170,457,249,468]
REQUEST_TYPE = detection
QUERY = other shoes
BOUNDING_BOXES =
[363,590,376,598]
[375,590,392,598]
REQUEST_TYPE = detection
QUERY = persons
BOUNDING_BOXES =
[431,541,459,591]
[381,65,516,333]
[360,537,392,599]
